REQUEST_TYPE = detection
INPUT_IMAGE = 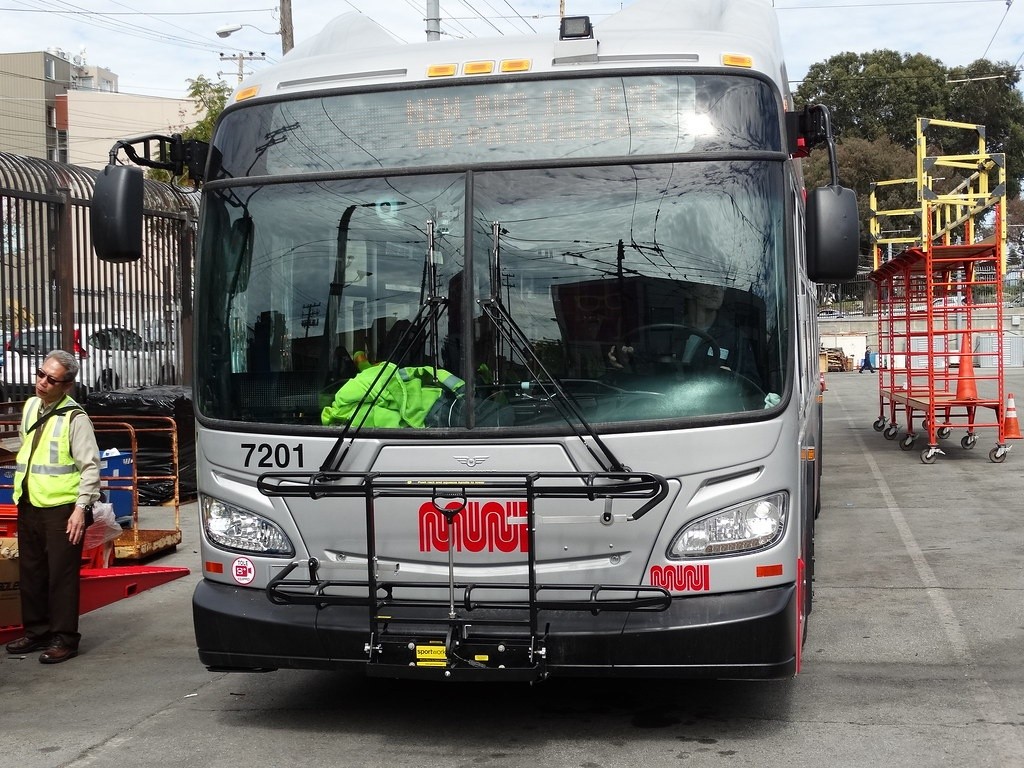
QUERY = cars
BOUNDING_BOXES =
[0,329,13,368]
[1,323,157,394]
[933,296,968,308]
[818,309,843,319]
[138,325,178,386]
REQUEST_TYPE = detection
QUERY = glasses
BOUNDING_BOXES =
[37,368,66,385]
[692,279,727,292]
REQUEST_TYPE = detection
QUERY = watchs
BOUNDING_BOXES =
[77,503,91,512]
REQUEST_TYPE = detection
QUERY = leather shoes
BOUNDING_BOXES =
[39,639,79,662]
[6,636,53,653]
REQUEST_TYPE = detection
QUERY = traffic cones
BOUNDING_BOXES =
[997,393,1024,439]
[949,332,981,400]
[820,371,829,392]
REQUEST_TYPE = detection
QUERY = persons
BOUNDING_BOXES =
[608,263,761,386]
[859,346,876,373]
[7,351,100,663]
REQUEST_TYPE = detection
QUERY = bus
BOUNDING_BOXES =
[90,12,861,687]
